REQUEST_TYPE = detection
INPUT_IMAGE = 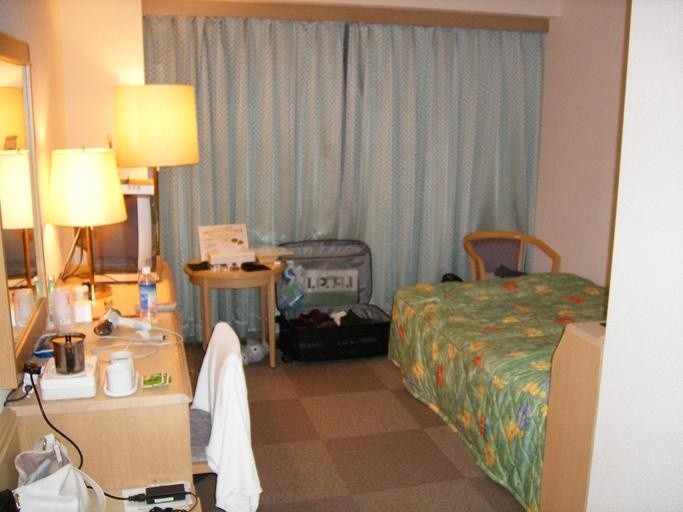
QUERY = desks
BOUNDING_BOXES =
[0,261,203,512]
[183,256,287,368]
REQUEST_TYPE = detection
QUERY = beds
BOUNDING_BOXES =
[389,273,606,512]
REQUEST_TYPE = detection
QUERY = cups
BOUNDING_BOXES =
[51,332,86,376]
[106,351,134,393]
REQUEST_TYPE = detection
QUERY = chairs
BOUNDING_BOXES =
[463,231,560,281]
[190,322,244,477]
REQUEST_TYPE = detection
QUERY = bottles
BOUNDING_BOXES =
[33,275,43,303]
[46,275,58,327]
[13,287,34,336]
[137,266,157,322]
[50,284,93,335]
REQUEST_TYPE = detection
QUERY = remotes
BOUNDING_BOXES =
[134,302,177,312]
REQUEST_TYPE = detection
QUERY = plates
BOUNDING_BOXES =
[104,369,139,397]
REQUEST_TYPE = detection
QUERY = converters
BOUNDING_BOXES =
[145,483,186,504]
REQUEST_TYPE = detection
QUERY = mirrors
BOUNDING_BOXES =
[0,30,50,390]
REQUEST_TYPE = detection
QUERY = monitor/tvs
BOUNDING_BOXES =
[90,193,163,283]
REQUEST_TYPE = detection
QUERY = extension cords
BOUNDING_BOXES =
[21,372,38,398]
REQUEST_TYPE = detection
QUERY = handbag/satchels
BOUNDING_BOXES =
[11,433,107,512]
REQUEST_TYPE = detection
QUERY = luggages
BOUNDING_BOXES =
[270,239,392,364]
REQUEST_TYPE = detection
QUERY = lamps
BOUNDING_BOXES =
[49,148,128,301]
[113,83,200,257]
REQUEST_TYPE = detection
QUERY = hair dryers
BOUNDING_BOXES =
[94,309,151,336]
